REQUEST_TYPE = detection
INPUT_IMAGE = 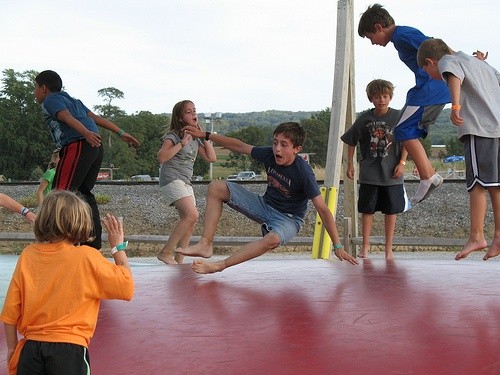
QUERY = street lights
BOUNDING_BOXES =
[204,112,222,180]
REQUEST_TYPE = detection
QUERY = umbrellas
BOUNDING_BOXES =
[443,155,464,163]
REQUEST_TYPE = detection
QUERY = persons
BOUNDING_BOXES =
[37,148,60,205]
[358,4,488,203]
[174,122,359,274]
[34,71,140,251]
[417,38,500,261]
[156,100,216,266]
[0,190,134,375]
[340,79,408,260]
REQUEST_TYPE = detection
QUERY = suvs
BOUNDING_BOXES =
[227,171,262,181]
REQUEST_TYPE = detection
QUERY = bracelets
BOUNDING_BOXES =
[400,160,406,165]
[21,207,29,216]
[110,241,128,258]
[119,130,124,135]
[205,131,211,141]
[334,244,343,249]
[451,106,461,111]
